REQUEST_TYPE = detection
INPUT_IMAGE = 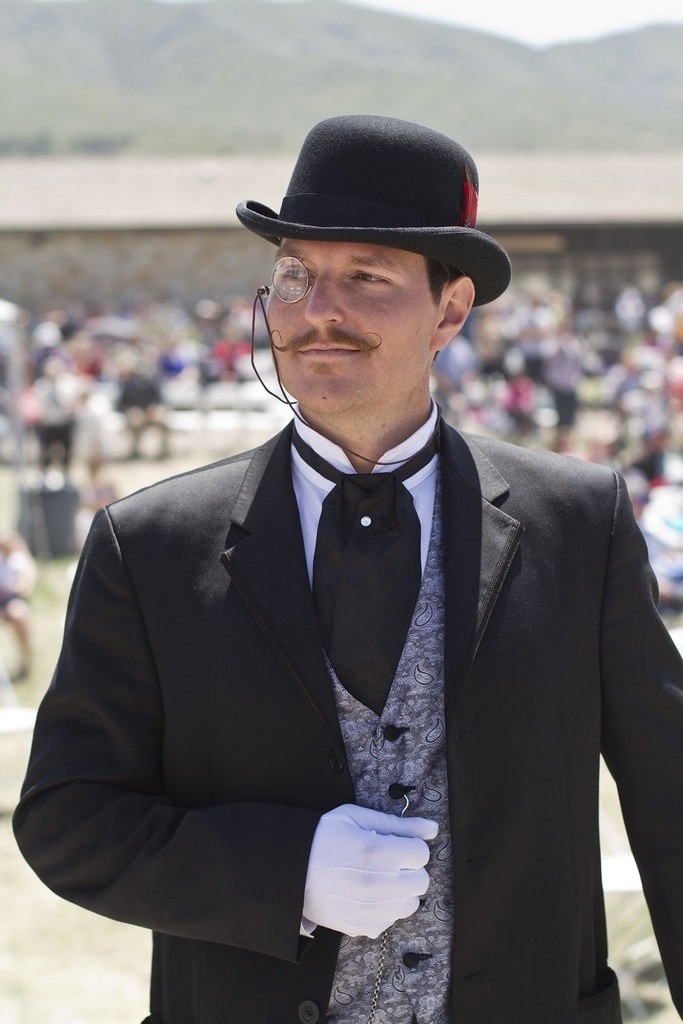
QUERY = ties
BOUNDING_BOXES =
[291,424,443,717]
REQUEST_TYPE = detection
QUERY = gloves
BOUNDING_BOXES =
[301,803,438,939]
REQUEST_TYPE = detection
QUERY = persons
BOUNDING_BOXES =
[433,278,683,611]
[11,114,682,1024]
[0,525,38,676]
[0,292,252,473]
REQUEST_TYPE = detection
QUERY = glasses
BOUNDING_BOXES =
[259,256,312,304]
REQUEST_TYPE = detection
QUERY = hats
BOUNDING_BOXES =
[236,115,512,308]
